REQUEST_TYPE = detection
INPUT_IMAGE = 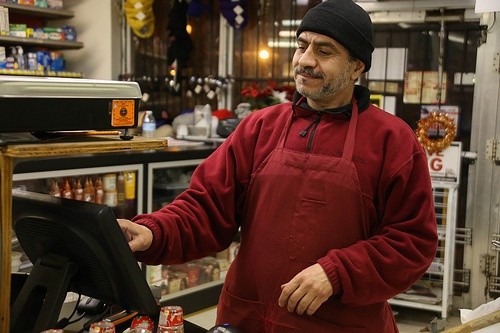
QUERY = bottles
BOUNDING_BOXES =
[49,175,104,204]
[117,172,125,204]
[142,111,156,137]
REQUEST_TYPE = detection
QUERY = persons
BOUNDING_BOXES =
[115,0,438,333]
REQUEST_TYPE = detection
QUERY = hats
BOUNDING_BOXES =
[296,0,374,73]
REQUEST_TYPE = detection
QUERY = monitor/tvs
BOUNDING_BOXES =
[10,190,160,318]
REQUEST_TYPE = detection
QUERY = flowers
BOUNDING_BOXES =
[240,81,295,109]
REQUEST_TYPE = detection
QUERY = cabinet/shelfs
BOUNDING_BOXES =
[0,140,240,333]
[0,0,86,78]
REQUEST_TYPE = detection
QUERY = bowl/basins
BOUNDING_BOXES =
[189,125,206,137]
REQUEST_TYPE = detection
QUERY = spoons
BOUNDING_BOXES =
[120,128,133,141]
